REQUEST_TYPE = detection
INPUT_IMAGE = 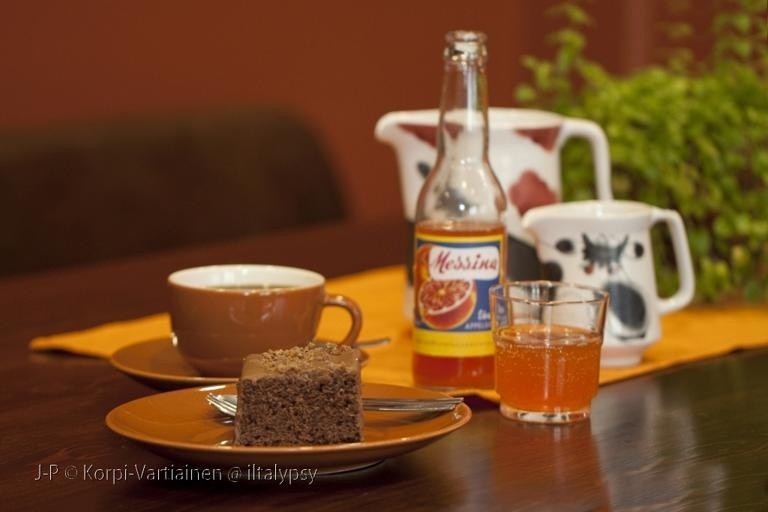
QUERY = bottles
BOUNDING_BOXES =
[415,27,509,401]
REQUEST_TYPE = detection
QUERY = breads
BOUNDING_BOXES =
[232,342,362,447]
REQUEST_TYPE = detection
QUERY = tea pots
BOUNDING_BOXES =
[521,195,696,370]
[375,105,614,343]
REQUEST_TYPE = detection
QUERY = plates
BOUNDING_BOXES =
[103,338,472,477]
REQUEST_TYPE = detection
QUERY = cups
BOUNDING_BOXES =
[490,281,606,427]
[165,265,363,377]
